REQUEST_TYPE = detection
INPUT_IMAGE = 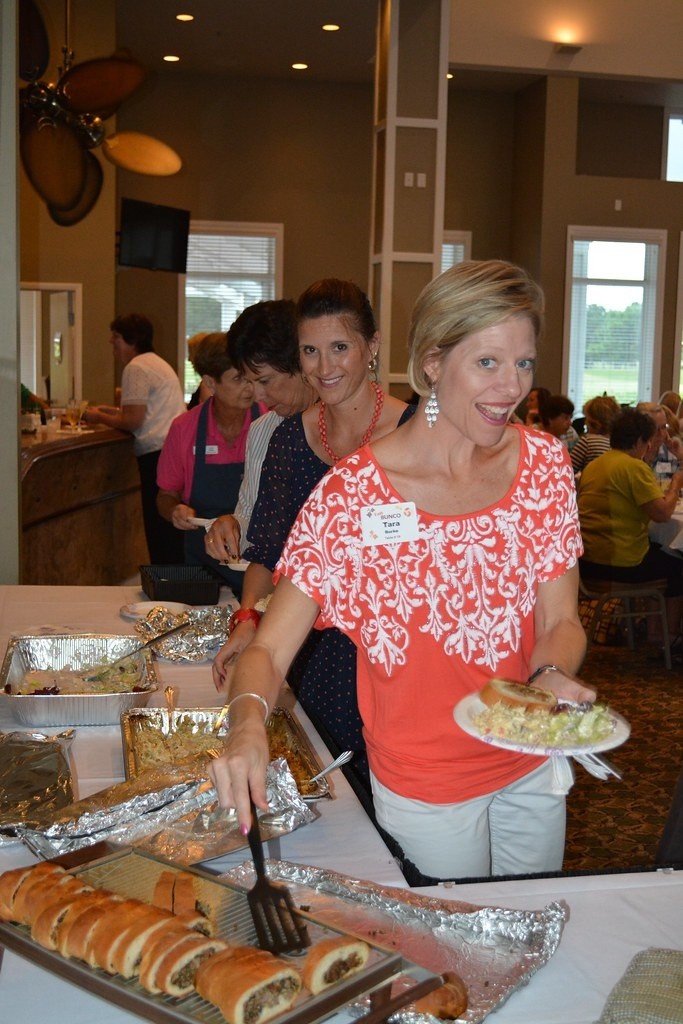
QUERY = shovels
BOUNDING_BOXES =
[247,779,312,953]
[76,621,194,680]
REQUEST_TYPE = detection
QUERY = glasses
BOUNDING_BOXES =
[655,423,669,432]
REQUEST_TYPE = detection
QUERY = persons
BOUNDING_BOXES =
[21,383,49,409]
[205,261,595,880]
[508,386,683,670]
[82,313,188,565]
[155,274,417,800]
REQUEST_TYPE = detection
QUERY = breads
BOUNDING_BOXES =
[0,864,468,1024]
[481,679,557,712]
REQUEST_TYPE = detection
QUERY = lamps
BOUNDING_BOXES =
[553,42,582,55]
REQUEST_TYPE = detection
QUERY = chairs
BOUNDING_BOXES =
[579,578,672,669]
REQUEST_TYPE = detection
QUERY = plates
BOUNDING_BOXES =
[218,558,250,572]
[187,517,209,526]
[451,690,632,756]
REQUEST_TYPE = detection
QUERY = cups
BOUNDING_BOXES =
[44,409,62,432]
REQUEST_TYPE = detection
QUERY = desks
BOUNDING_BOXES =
[646,486,683,560]
[19,416,150,586]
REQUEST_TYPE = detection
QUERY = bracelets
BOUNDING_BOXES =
[670,487,679,496]
[226,693,270,725]
[526,664,559,687]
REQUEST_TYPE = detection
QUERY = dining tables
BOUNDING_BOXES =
[0,584,683,1024]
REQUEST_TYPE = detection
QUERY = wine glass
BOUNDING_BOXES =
[66,400,89,436]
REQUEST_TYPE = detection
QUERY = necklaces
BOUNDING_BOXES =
[318,380,386,464]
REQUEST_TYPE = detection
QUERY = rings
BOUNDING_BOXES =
[210,538,213,543]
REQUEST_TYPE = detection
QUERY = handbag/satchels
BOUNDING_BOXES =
[578,596,648,642]
